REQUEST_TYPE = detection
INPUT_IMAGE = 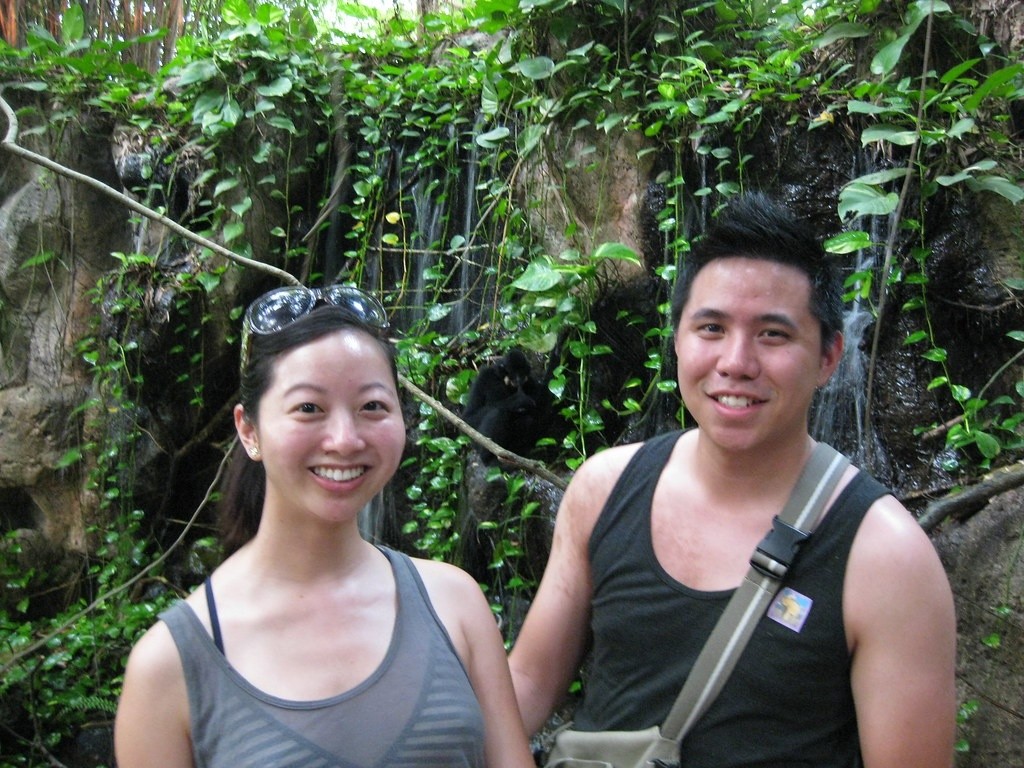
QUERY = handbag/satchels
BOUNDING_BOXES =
[542,725,680,768]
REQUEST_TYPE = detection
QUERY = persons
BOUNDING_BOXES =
[507,191,958,768]
[115,284,542,768]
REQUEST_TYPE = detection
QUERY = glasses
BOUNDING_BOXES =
[240,285,390,399]
